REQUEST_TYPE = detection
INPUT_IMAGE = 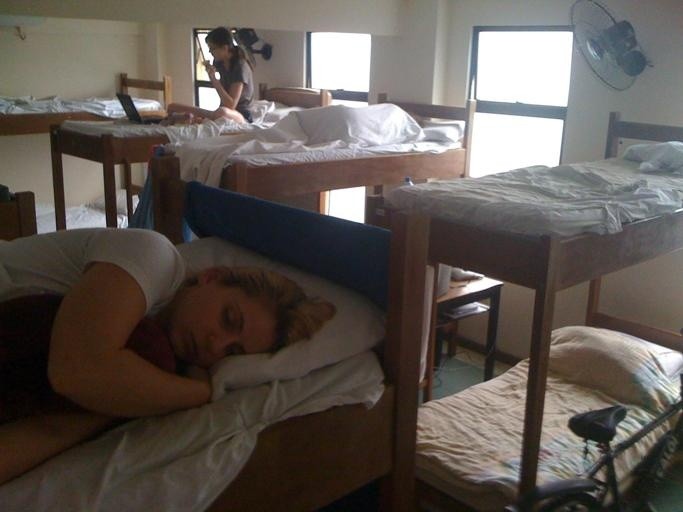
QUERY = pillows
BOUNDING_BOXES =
[255,101,307,123]
[420,119,464,144]
[599,325,682,382]
[84,188,140,216]
[174,237,388,402]
[622,143,653,161]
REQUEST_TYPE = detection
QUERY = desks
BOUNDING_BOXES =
[432,270,505,383]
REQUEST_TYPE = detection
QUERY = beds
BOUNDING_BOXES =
[163,91,477,199]
[2,151,432,510]
[36,182,145,236]
[418,311,682,509]
[2,71,174,137]
[388,110,682,294]
[48,81,334,165]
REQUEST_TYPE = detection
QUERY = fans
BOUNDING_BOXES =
[569,0,655,92]
[231,27,272,69]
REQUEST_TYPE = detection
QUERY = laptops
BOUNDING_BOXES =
[117,93,165,124]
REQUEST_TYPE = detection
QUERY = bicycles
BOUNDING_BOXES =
[505,373,683,512]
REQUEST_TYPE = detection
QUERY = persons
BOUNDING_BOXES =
[0,226,335,488]
[166,27,254,125]
